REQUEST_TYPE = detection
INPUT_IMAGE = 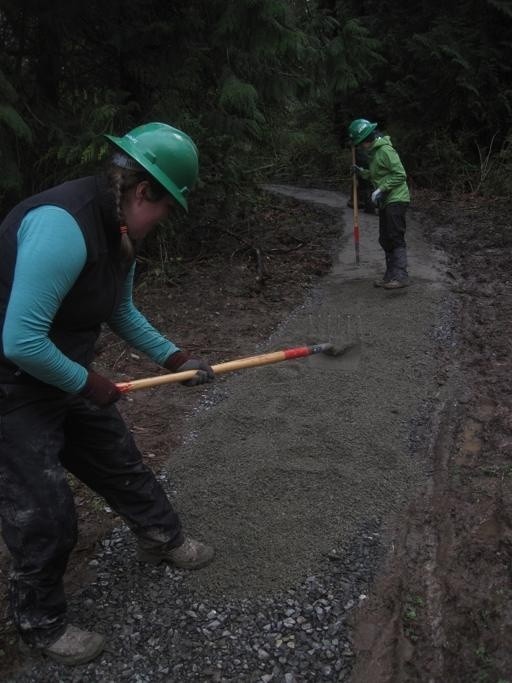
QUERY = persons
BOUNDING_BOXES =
[346,115,413,290]
[1,115,220,659]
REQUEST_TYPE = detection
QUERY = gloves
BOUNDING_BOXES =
[370,187,382,207]
[349,164,363,178]
[164,348,215,388]
[76,368,122,411]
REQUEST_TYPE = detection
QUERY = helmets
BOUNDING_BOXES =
[101,121,202,217]
[347,118,378,147]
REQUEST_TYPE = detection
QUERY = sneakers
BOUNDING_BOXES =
[133,536,215,572]
[373,276,409,289]
[41,622,106,666]
